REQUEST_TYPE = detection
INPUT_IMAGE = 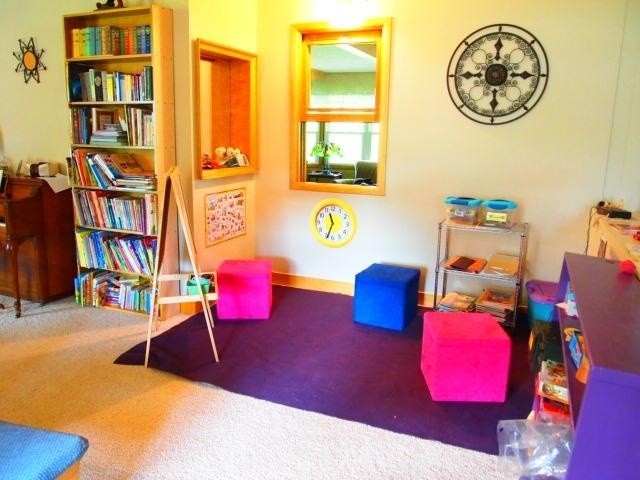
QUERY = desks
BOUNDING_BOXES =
[308,172,343,184]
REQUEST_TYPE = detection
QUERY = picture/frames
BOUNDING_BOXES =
[92,106,117,132]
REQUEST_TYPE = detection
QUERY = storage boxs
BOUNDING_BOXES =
[525,279,559,330]
[480,198,519,229]
[443,195,483,227]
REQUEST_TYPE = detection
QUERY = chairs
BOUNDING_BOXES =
[0,185,48,318]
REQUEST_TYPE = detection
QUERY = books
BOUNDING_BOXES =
[72,190,158,236]
[78,66,152,101]
[71,24,151,55]
[475,289,514,324]
[66,147,157,190]
[71,105,154,146]
[75,230,157,277]
[437,292,475,312]
[485,253,519,276]
[74,270,159,315]
[611,222,639,236]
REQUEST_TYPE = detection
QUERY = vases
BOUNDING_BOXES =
[322,158,330,174]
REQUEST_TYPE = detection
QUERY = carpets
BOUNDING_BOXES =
[113,282,541,465]
[0,293,523,480]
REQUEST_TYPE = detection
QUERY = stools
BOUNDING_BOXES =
[353,263,420,331]
[420,311,511,402]
[216,259,272,320]
[0,420,89,480]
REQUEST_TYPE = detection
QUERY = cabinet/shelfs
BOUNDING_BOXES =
[533,253,640,480]
[584,207,640,279]
[433,218,529,338]
[0,173,77,306]
[62,4,183,320]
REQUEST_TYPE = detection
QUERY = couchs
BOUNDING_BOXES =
[330,160,377,187]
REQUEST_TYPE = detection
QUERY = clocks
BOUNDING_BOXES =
[310,198,357,249]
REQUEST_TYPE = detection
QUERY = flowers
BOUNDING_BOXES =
[310,140,344,160]
